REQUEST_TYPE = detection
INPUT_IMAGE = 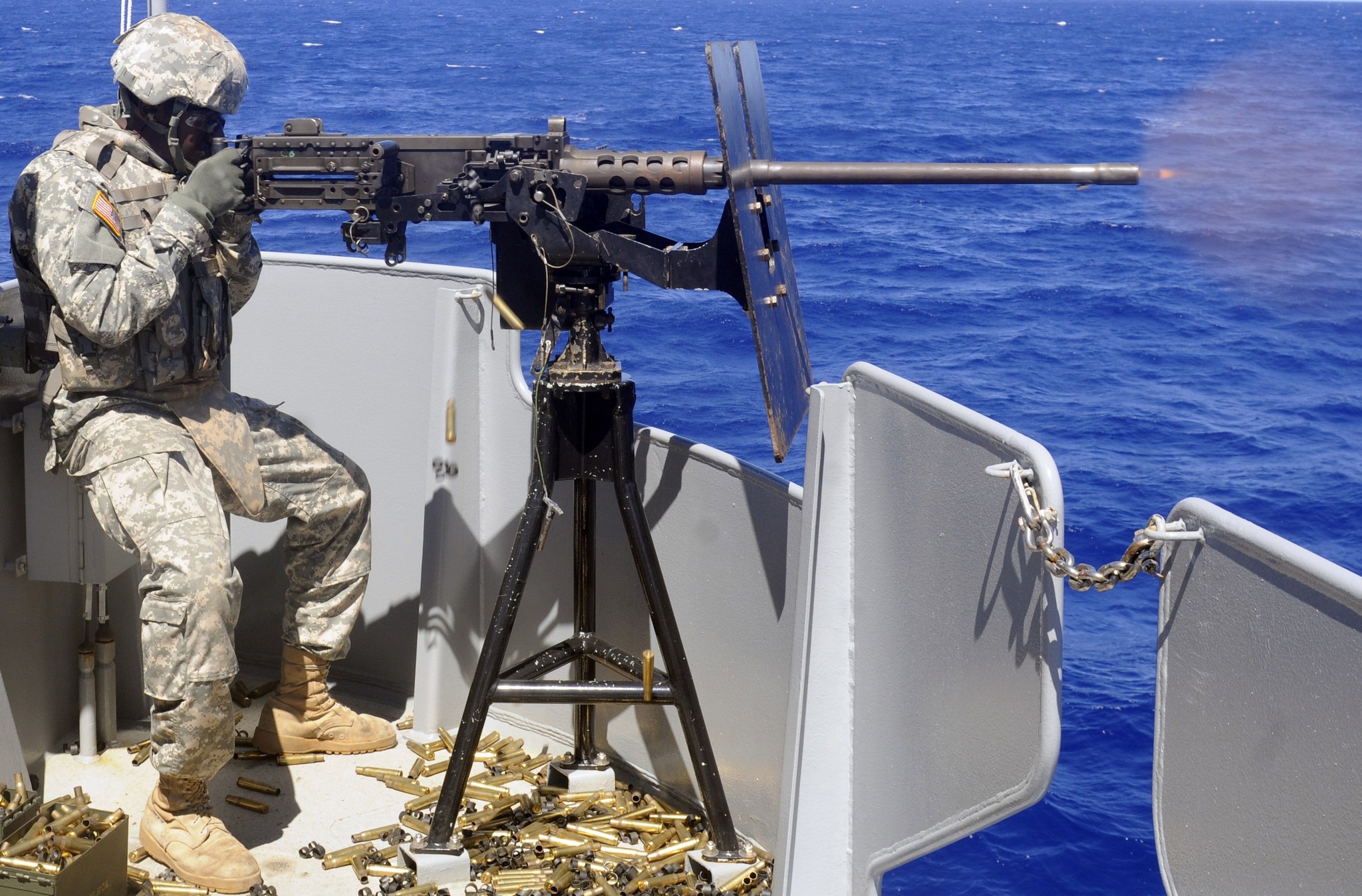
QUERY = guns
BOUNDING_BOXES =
[209,38,1142,857]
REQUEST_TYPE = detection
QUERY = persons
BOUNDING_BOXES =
[10,12,397,894]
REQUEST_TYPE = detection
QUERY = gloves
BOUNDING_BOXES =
[172,146,245,229]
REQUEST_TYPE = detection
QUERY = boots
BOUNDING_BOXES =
[140,773,264,894]
[251,643,397,754]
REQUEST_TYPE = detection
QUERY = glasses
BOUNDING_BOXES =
[180,112,222,134]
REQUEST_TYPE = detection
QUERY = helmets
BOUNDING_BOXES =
[111,12,248,115]
